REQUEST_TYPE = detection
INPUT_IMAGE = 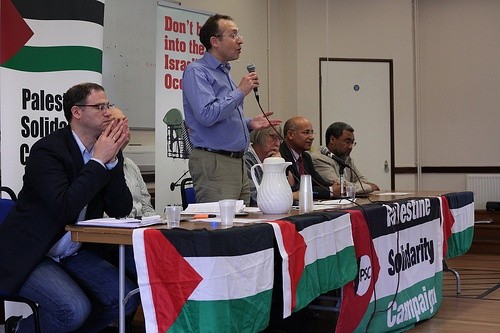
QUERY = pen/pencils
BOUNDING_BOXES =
[339,199,342,203]
[313,191,318,194]
[116,216,142,219]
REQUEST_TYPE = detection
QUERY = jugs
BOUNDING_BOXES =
[250,157,294,214]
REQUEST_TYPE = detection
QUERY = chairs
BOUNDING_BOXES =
[0,186,40,333]
[181,177,196,210]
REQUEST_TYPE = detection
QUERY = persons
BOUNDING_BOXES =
[245,124,300,206]
[0,83,137,333]
[107,106,159,283]
[182,14,282,203]
[311,122,380,194]
[270,116,340,199]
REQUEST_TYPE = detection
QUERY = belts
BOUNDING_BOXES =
[194,146,244,158]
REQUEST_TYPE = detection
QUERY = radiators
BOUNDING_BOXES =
[465,174,500,209]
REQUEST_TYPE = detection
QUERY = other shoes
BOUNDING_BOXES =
[5,315,23,333]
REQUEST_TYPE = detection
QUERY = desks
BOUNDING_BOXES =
[65,191,474,332]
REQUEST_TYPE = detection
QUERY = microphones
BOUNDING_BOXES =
[246,62,258,92]
[320,146,350,169]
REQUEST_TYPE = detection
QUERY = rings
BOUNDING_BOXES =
[113,138,115,140]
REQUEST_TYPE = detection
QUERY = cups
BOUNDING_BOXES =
[166,206,181,228]
[298,174,314,213]
[346,185,356,199]
[219,199,236,226]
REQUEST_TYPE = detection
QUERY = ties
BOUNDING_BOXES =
[298,156,304,176]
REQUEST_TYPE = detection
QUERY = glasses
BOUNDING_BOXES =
[344,139,356,146]
[72,104,115,111]
[269,134,283,144]
[301,129,314,134]
[214,33,242,40]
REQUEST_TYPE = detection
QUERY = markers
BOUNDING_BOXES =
[194,214,216,218]
